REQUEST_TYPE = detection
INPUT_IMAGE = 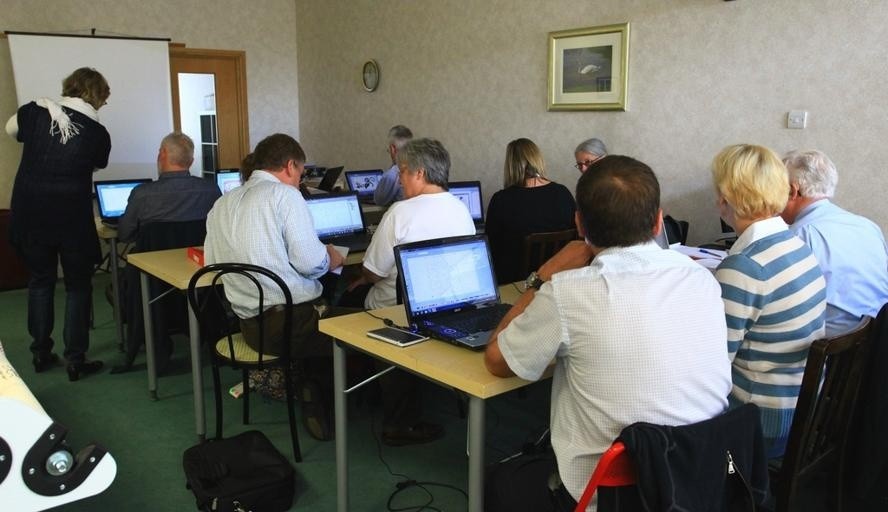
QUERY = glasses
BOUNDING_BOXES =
[574,156,601,169]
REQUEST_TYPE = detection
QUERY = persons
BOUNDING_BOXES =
[484,137,578,286]
[373,124,415,206]
[484,153,732,512]
[574,138,607,175]
[713,144,828,459]
[117,132,222,378]
[204,134,345,442]
[5,67,112,381]
[778,148,888,338]
[336,138,478,309]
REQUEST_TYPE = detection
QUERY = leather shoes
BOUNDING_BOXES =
[68,360,102,381]
[32,353,57,372]
[299,378,329,440]
[384,423,444,446]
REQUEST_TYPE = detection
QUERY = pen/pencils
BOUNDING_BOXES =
[699,250,721,257]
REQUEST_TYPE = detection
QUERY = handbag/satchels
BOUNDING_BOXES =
[248,361,298,402]
[183,430,295,512]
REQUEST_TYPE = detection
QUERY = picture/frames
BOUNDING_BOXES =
[546,21,631,112]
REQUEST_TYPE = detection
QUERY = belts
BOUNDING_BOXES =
[255,304,285,320]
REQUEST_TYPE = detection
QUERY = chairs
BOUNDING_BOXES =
[94,191,888,512]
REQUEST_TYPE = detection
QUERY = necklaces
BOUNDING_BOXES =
[736,215,773,237]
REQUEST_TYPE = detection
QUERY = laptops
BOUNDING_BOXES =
[303,190,373,254]
[393,234,514,351]
[447,181,487,230]
[305,166,344,195]
[94,179,153,229]
[344,169,384,205]
[214,168,246,196]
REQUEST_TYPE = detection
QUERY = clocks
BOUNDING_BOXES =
[361,59,379,92]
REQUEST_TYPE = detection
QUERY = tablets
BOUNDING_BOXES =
[366,327,426,348]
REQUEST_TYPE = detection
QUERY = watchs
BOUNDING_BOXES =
[525,270,544,291]
